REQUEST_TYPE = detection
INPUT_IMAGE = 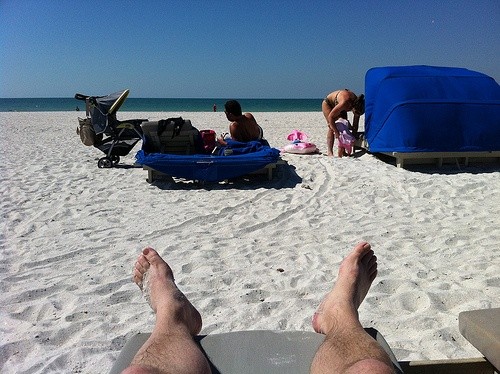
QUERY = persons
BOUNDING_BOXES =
[217,100,263,146]
[328,110,357,158]
[322,89,365,159]
[121,241,403,374]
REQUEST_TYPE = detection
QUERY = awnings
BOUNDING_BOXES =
[364,65,500,153]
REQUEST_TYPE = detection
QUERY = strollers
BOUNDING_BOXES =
[73,87,149,168]
